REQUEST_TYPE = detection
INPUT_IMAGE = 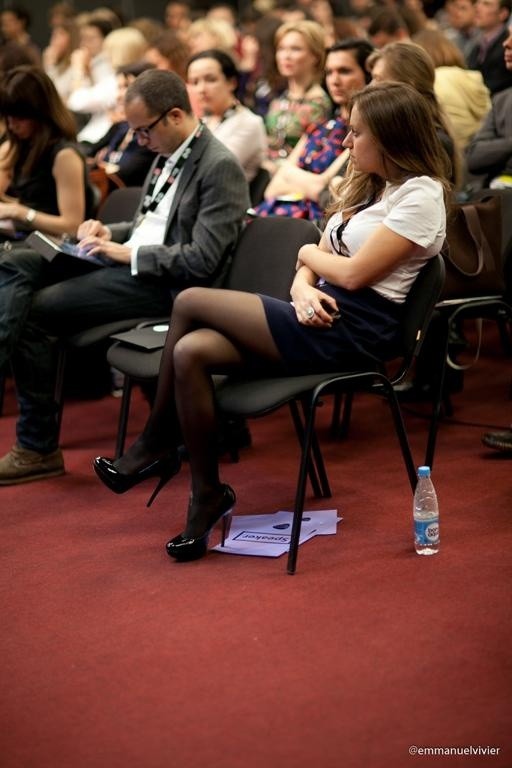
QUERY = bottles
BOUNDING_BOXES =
[411,465,441,557]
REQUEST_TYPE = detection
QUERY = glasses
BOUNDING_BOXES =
[131,106,175,138]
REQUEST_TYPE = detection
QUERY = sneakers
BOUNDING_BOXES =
[0,444,68,487]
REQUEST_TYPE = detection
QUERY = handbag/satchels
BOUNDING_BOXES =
[438,194,510,307]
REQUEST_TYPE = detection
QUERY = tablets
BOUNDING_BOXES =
[34,227,106,267]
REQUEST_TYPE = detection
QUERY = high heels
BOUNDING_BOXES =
[166,482,237,563]
[92,449,183,508]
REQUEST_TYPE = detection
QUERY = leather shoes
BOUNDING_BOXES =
[383,363,464,400]
[481,425,511,457]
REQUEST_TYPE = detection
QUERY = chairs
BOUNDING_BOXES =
[12,149,512,575]
